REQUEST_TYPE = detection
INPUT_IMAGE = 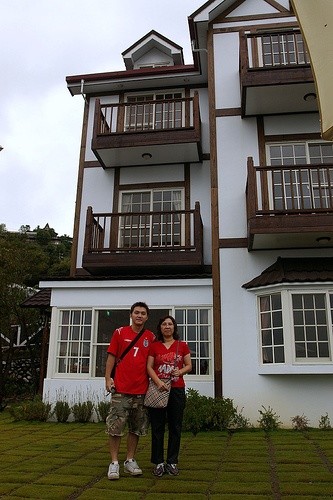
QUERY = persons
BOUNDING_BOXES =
[105,302,156,478]
[146,316,192,476]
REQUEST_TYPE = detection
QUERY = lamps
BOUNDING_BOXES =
[141,153,152,160]
[315,236,331,243]
[303,92,317,102]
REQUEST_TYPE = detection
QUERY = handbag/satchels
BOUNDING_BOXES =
[144,377,172,408]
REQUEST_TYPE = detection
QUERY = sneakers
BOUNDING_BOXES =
[108,463,119,480]
[123,458,143,475]
[152,463,179,477]
